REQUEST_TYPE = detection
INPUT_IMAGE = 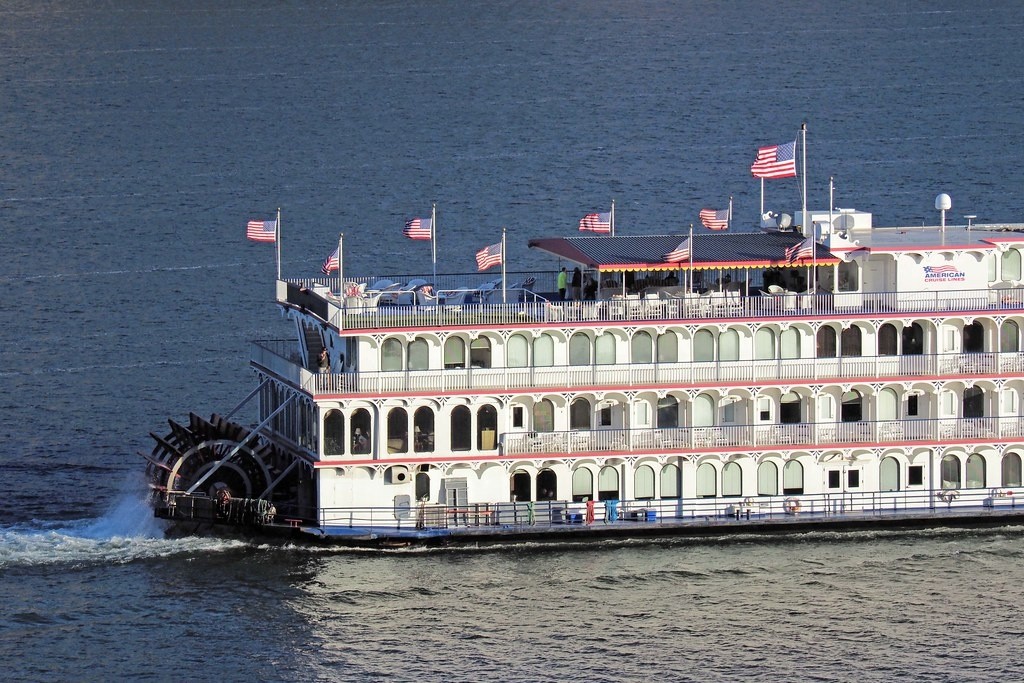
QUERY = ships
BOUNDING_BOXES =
[138,116,1024,548]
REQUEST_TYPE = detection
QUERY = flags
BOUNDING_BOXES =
[661,237,690,262]
[579,211,611,232]
[476,242,502,271]
[246,220,276,242]
[402,218,432,239]
[700,208,729,230]
[751,140,796,178]
[786,236,814,264]
[321,247,339,275]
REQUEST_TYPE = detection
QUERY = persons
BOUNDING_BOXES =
[557,267,567,300]
[572,267,581,307]
[584,270,679,305]
[317,347,329,390]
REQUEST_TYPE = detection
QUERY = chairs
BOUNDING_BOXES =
[324,275,539,316]
[523,428,729,452]
[326,359,356,390]
[547,271,816,323]
[958,353,1024,374]
[938,416,1024,439]
[755,422,903,447]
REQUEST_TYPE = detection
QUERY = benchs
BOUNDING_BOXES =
[447,510,502,526]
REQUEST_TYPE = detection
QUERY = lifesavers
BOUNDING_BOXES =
[784,497,802,514]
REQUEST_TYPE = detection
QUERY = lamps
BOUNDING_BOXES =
[414,426,421,436]
[355,428,361,438]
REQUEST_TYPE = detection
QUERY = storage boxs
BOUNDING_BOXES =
[646,509,657,522]
